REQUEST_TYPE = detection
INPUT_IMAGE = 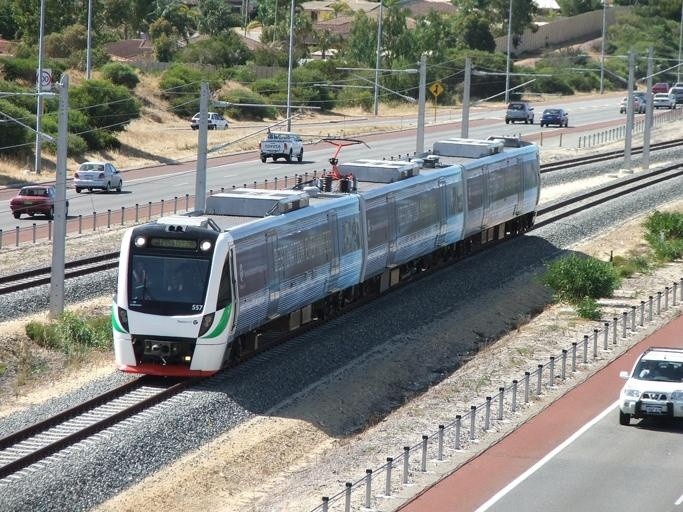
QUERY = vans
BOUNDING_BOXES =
[504,103,534,124]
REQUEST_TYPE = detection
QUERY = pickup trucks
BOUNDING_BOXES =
[259,131,303,163]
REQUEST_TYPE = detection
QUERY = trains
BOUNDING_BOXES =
[112,135,540,377]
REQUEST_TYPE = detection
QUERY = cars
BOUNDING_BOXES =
[618,348,683,426]
[540,109,569,127]
[619,82,683,114]
[191,111,229,130]
[73,161,123,192]
[9,185,69,220]
